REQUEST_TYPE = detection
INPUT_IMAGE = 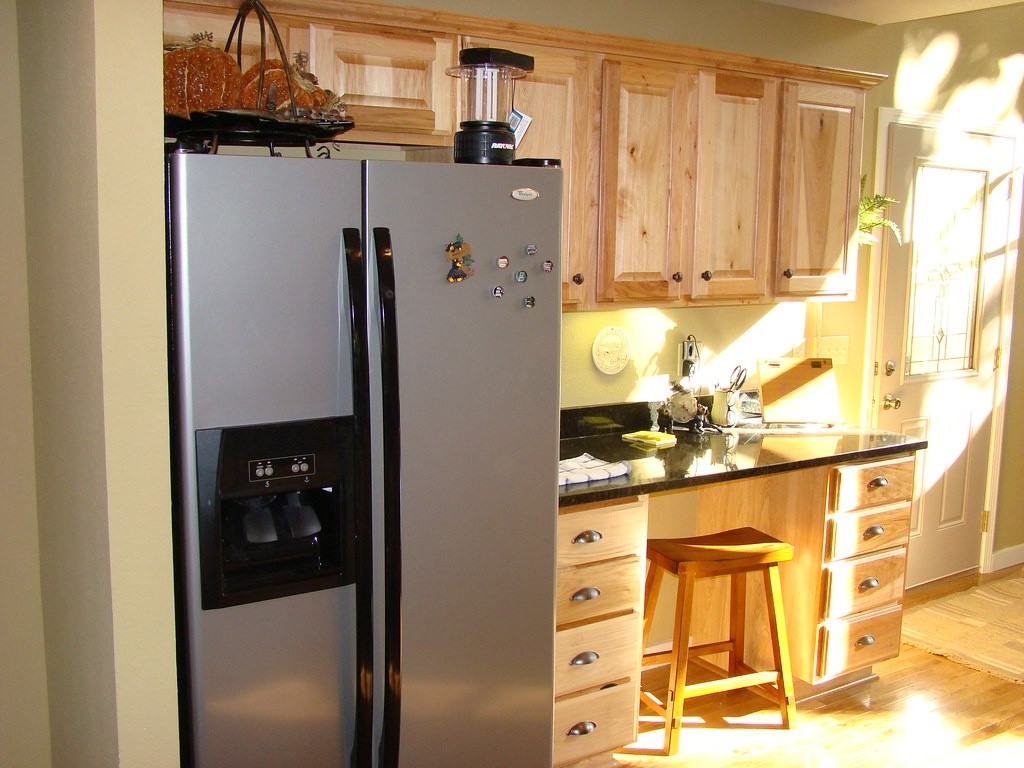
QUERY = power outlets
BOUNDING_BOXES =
[677,341,703,377]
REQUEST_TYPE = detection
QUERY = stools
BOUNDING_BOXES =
[638,527,799,757]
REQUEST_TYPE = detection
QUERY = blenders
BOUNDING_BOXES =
[443,47,535,167]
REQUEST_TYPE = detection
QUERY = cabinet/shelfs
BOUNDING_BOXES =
[591,31,776,312]
[687,452,917,711]
[546,490,656,768]
[771,61,889,305]
[405,14,598,313]
[163,0,457,152]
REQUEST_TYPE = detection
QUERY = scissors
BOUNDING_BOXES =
[729,365,747,389]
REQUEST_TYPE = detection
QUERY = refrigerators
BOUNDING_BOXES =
[162,154,565,768]
